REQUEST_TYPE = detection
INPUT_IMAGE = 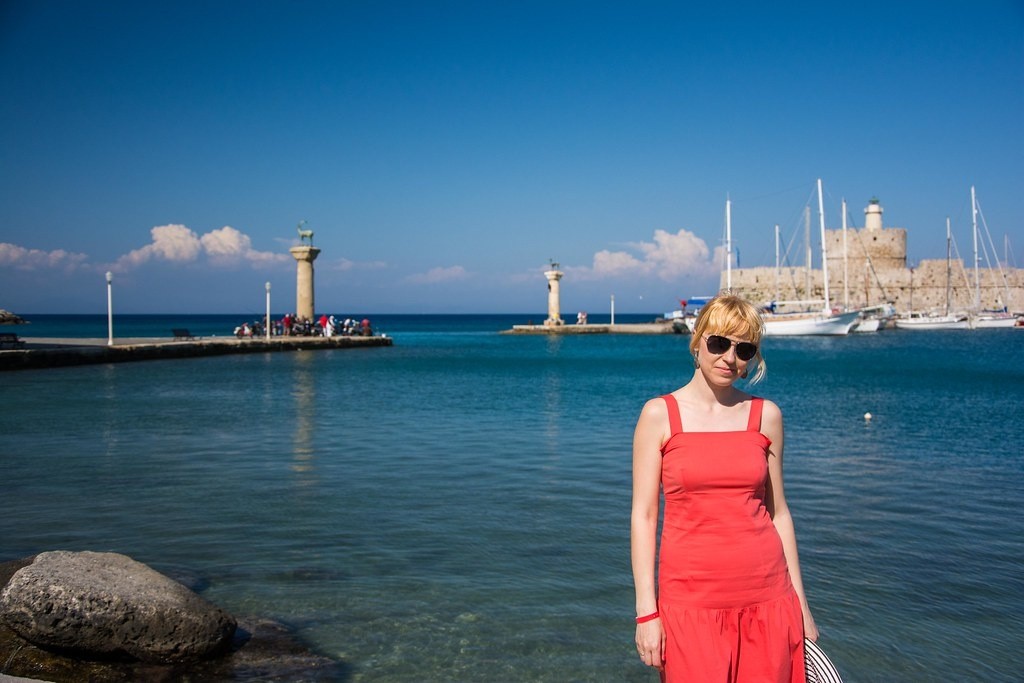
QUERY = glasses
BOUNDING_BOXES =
[701,334,757,361]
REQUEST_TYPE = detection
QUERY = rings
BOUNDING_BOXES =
[640,651,645,656]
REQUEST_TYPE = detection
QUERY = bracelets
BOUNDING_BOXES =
[636,611,659,624]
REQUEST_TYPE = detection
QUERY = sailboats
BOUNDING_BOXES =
[896,185,1022,329]
[674,178,893,336]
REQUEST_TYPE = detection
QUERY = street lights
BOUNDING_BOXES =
[104,270,116,347]
[264,280,272,342]
[611,292,616,324]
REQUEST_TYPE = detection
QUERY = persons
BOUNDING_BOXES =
[631,290,819,683]
[233,313,373,339]
